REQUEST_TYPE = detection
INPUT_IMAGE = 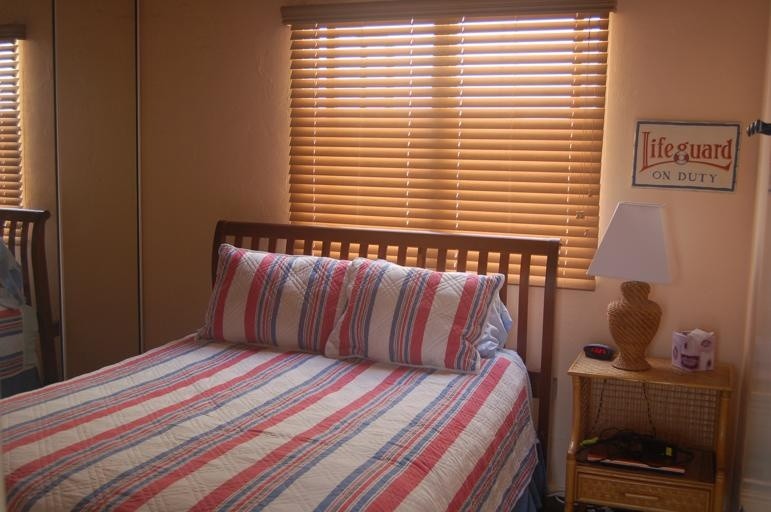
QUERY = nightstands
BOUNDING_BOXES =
[566,347,741,511]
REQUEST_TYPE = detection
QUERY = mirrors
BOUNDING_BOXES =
[0,1,61,403]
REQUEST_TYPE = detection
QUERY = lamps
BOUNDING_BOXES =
[583,201,673,374]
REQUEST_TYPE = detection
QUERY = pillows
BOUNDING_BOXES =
[323,255,507,377]
[193,241,352,356]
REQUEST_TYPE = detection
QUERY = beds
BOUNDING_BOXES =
[1,217,564,512]
[1,206,59,386]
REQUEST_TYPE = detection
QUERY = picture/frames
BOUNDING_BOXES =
[631,119,743,193]
